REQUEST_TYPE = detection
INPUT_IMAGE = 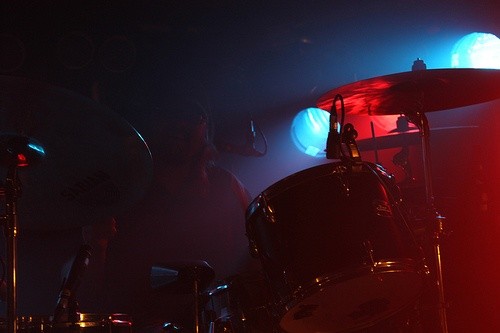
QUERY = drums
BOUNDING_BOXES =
[241,160,431,332]
[4,315,136,333]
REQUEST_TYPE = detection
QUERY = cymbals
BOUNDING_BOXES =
[1,74,156,234]
[315,66,500,116]
[324,124,483,160]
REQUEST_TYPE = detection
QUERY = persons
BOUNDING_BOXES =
[97,74,253,311]
